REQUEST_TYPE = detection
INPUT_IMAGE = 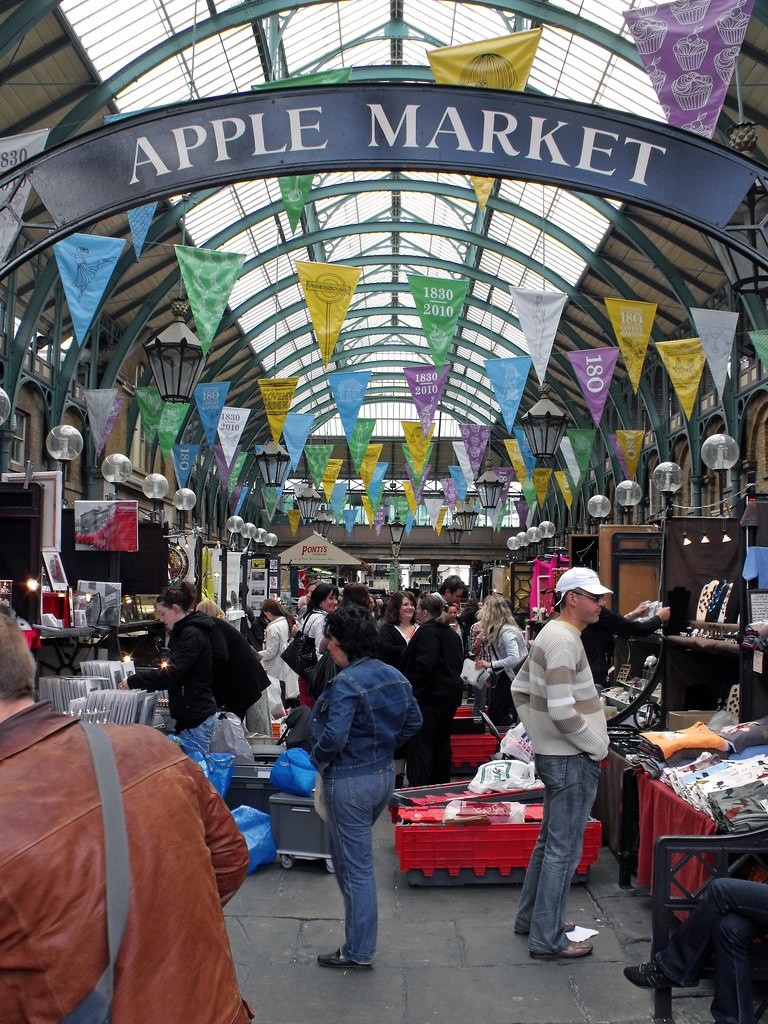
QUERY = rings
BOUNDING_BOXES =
[120,686,122,688]
[119,682,123,684]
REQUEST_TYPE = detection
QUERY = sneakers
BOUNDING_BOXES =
[623,961,700,989]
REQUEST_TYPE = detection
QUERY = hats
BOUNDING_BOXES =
[555,567,613,606]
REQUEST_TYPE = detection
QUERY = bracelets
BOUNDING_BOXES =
[629,612,634,621]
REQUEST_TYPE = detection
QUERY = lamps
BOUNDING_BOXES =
[452,495,478,532]
[445,519,464,546]
[137,191,215,403]
[253,224,291,486]
[682,519,732,545]
[386,511,416,571]
[295,476,333,538]
[472,288,505,510]
[519,380,570,460]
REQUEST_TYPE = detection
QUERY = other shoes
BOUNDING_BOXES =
[514,921,575,935]
[529,941,593,958]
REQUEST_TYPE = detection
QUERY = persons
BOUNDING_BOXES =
[403,595,465,787]
[117,583,217,756]
[1,608,255,1024]
[306,604,425,968]
[194,600,271,738]
[510,567,614,957]
[243,574,494,717]
[581,605,671,700]
[623,877,768,1023]
[474,593,529,726]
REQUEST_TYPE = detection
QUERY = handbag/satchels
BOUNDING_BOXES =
[467,721,537,795]
[167,734,236,799]
[270,748,318,798]
[210,711,255,765]
[280,612,323,681]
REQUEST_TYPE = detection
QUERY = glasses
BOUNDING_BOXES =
[572,591,605,602]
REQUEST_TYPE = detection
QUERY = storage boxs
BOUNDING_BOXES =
[269,790,332,856]
[668,711,718,731]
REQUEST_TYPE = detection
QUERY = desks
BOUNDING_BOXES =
[589,746,768,924]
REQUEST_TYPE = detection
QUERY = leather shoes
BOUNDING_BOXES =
[317,947,372,969]
